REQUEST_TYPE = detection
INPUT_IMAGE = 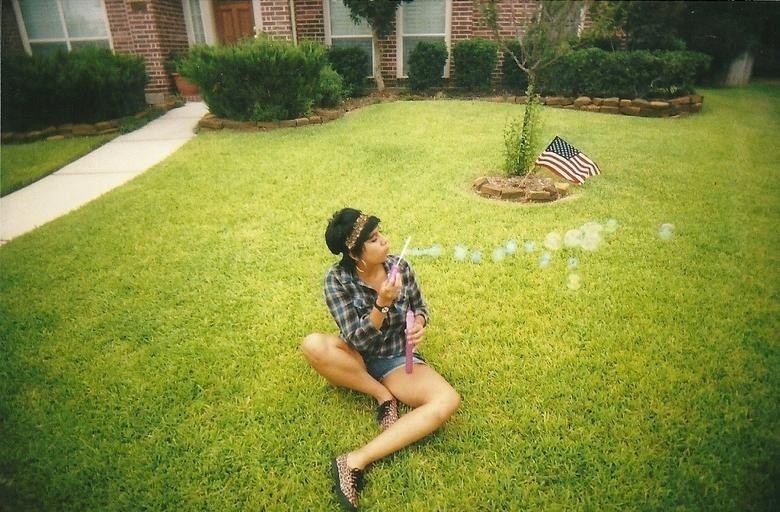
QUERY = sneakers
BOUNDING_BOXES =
[378,398,398,429]
[332,454,361,509]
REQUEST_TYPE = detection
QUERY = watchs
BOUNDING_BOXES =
[373,297,392,316]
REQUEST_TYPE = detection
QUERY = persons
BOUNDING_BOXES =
[300,207,461,512]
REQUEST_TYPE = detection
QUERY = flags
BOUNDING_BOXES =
[534,136,600,185]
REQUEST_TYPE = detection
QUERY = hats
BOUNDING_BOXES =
[325,208,379,255]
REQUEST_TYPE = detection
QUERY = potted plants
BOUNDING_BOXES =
[163,49,201,94]
